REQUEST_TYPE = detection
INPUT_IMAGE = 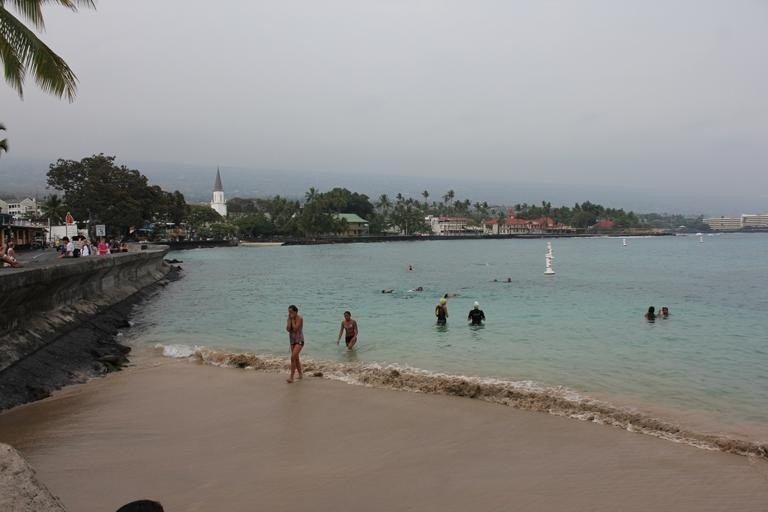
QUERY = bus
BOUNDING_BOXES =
[151,222,187,234]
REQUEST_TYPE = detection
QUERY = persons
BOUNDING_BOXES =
[285,304,305,384]
[412,287,423,291]
[48,236,130,258]
[335,310,358,350]
[381,288,394,294]
[466,301,486,326]
[0,237,25,269]
[116,499,163,512]
[493,275,512,283]
[409,265,415,270]
[643,305,671,320]
[433,293,449,327]
